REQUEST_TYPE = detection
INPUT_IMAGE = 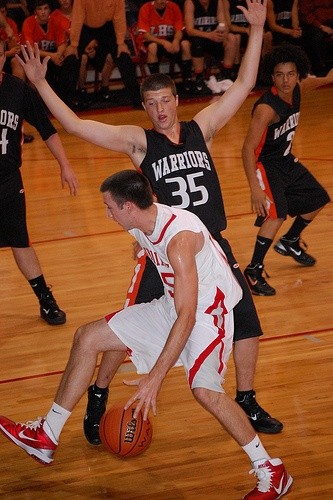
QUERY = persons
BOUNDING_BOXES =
[15,0,284,447]
[0,38,79,324]
[241,45,333,297]
[0,0,333,142]
[0,170,296,500]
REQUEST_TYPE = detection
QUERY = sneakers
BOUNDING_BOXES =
[234,393,284,434]
[242,264,277,297]
[39,294,67,325]
[0,415,58,466]
[76,84,143,114]
[22,135,34,144]
[177,71,238,102]
[273,235,318,266]
[82,384,110,446]
[242,457,295,499]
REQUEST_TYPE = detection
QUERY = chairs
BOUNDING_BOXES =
[74,1,225,112]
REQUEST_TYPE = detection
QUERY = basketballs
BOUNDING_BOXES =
[99,404,154,457]
